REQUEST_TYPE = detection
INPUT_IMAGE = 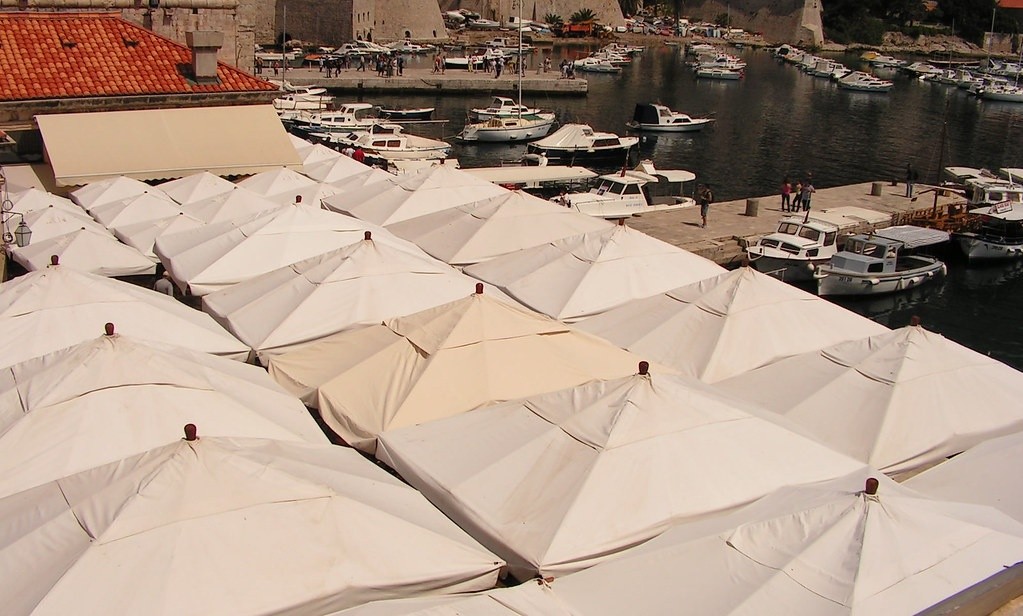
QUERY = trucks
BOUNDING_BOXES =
[554,22,590,38]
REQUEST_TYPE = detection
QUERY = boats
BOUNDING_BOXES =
[379,106,434,119]
[459,165,598,199]
[455,114,559,141]
[772,42,893,91]
[269,81,454,158]
[862,51,1023,102]
[625,105,718,130]
[744,206,893,282]
[469,98,542,123]
[690,40,746,80]
[553,164,698,217]
[487,36,535,50]
[942,166,1022,200]
[524,125,643,158]
[574,41,648,75]
[445,49,531,67]
[950,200,1023,260]
[333,40,434,57]
[816,238,952,297]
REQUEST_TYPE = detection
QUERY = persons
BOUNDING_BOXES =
[697,182,712,228]
[559,59,577,80]
[780,176,816,213]
[467,54,529,79]
[153,270,174,296]
[433,54,447,74]
[305,134,366,165]
[559,187,571,207]
[905,164,918,198]
[256,57,292,77]
[544,57,552,73]
[318,51,409,79]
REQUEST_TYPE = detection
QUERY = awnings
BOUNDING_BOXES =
[32,103,308,191]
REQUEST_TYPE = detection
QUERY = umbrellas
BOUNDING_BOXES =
[297,143,343,165]
[297,156,372,182]
[0,167,1023,616]
